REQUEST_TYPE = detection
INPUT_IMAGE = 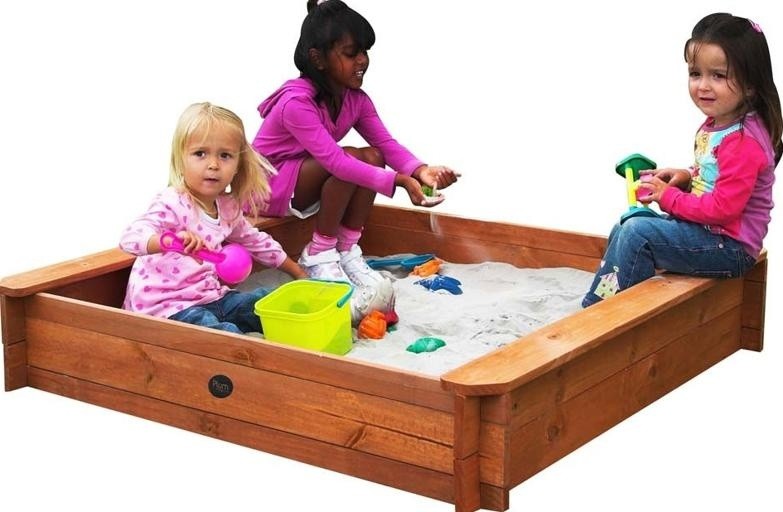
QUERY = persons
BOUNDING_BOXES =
[119,101,397,338]
[238,0,462,290]
[579,14,783,309]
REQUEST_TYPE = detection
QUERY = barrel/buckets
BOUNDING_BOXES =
[252,275,356,358]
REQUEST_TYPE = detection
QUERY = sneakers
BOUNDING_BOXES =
[351,278,394,324]
[339,243,385,287]
[298,245,360,298]
